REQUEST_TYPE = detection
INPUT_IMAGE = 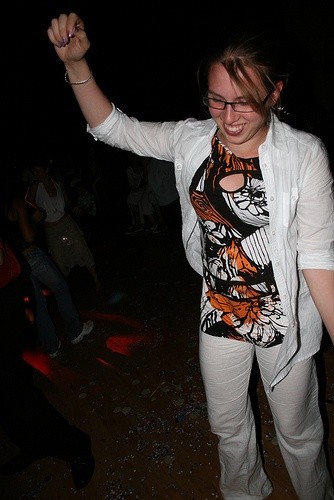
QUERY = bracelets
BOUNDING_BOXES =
[65,70,92,84]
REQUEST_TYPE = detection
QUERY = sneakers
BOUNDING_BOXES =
[48,320,94,359]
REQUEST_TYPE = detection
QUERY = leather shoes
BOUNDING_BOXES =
[72,436,95,490]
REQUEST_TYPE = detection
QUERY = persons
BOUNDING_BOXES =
[0,143,179,493]
[47,12,334,500]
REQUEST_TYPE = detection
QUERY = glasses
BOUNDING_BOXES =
[201,95,272,113]
